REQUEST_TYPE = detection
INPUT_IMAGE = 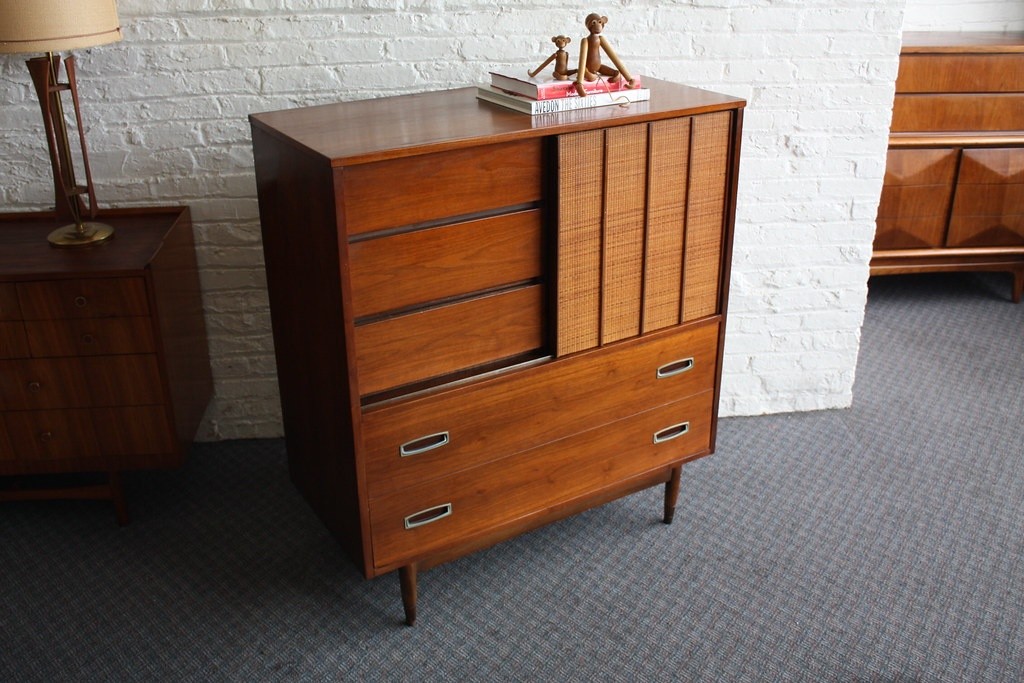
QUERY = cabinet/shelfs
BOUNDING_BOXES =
[868,31,1024,304]
[246,75,747,628]
[0,204,215,529]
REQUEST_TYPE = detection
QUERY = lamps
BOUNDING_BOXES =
[0,0,125,251]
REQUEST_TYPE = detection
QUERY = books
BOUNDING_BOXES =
[474,63,650,116]
